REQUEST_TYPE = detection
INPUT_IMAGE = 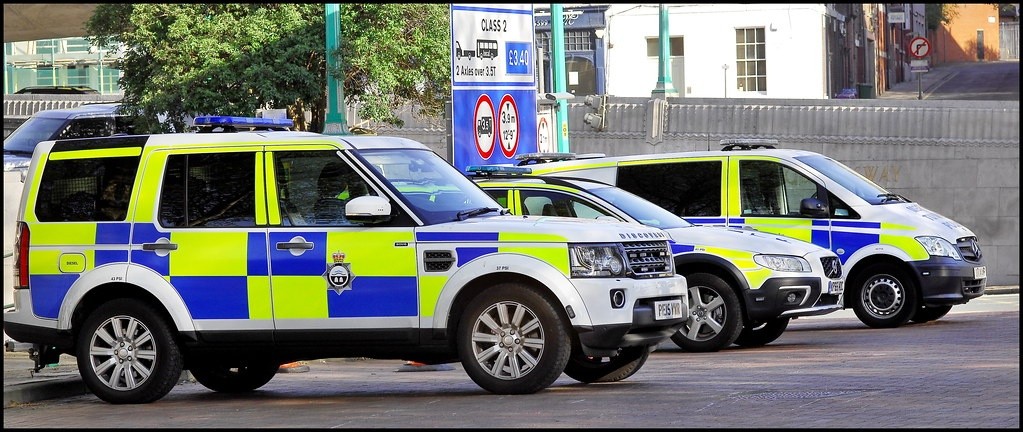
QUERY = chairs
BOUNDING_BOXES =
[543,203,559,217]
[315,163,350,220]
[279,178,309,225]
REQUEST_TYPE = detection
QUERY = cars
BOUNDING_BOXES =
[312,162,845,352]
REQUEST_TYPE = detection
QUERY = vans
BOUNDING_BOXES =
[1,103,189,322]
[514,135,986,331]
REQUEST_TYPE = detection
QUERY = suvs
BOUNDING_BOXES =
[1,114,691,396]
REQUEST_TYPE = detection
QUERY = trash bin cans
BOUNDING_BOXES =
[856,82,874,99]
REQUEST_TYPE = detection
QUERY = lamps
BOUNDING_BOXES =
[585,94,603,110]
[582,112,604,130]
